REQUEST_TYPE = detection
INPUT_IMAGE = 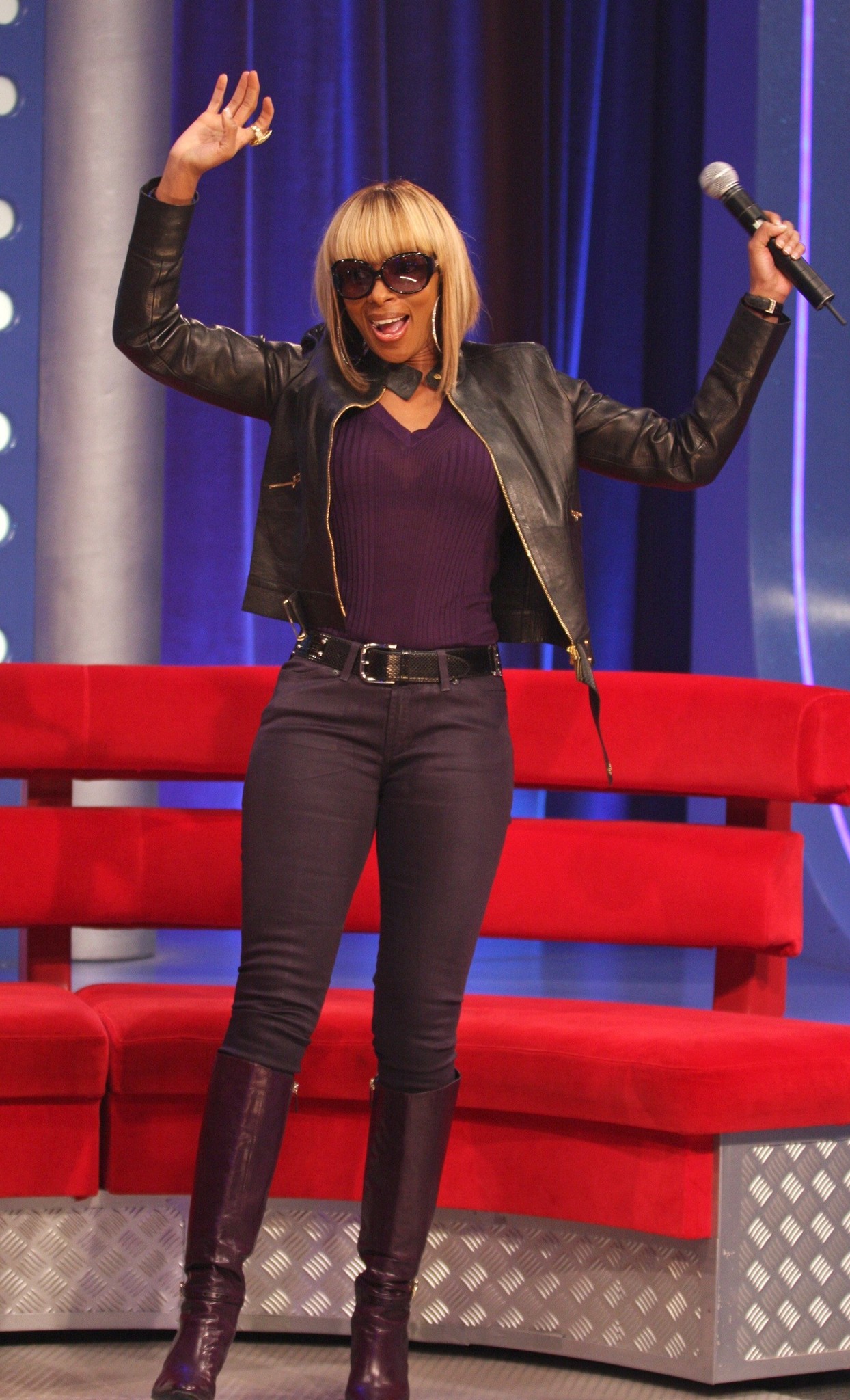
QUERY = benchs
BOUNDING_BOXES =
[0,662,850,1387]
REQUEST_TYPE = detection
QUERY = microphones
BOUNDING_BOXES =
[698,161,846,325]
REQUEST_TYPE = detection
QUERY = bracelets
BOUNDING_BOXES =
[744,291,783,317]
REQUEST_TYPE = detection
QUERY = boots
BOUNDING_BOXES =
[344,1066,462,1400]
[149,1051,300,1400]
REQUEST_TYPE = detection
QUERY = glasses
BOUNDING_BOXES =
[331,251,439,301]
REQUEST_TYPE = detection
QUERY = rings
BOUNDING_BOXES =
[251,125,273,146]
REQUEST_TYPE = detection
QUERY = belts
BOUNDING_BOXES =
[291,632,500,685]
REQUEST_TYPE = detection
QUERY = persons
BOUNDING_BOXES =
[61,67,804,1400]
